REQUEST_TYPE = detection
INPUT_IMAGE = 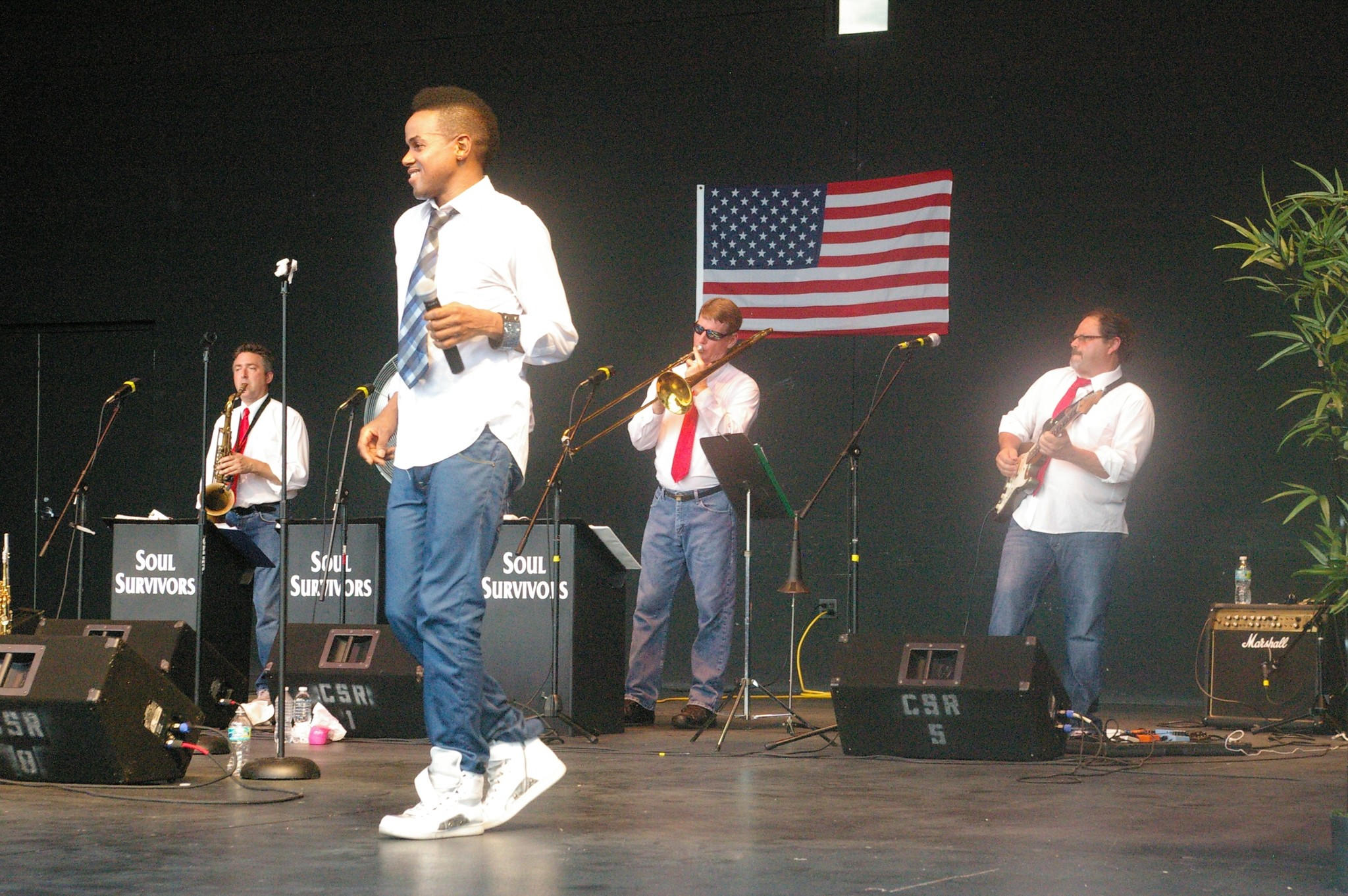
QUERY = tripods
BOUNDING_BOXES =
[691,488,839,753]
[521,475,599,745]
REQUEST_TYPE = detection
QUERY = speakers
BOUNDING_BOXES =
[829,634,1073,763]
[264,621,427,739]
[33,618,250,728]
[1201,602,1338,733]
[1,634,203,784]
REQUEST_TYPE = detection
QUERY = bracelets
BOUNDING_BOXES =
[487,312,521,352]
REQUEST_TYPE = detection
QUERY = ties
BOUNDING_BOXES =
[396,207,461,389]
[670,390,699,483]
[1032,376,1092,497]
[221,408,250,504]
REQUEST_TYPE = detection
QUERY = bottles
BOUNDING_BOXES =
[1233,556,1252,605]
[226,707,251,776]
[292,685,312,743]
[274,685,293,743]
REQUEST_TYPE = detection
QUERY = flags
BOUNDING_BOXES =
[692,168,954,341]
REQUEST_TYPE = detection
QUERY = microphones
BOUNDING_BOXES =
[898,333,941,350]
[580,365,618,386]
[340,383,375,408]
[414,277,466,375]
[106,378,142,403]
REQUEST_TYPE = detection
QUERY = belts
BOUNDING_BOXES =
[235,501,280,516]
[659,483,723,502]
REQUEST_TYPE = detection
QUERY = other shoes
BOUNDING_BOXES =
[252,689,273,725]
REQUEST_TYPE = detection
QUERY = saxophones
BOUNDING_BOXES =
[197,384,250,525]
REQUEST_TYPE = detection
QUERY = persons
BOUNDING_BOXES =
[353,83,577,843]
[624,298,761,733]
[983,304,1156,716]
[195,342,310,726]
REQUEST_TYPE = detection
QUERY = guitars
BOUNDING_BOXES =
[993,388,1110,517]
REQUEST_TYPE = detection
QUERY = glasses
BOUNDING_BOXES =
[1070,334,1115,343]
[694,320,739,341]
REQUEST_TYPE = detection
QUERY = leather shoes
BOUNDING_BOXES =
[671,704,717,729]
[624,699,656,726]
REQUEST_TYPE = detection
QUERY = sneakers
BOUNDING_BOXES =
[378,746,484,840]
[482,738,568,829]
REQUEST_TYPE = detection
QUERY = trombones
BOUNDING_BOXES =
[560,327,781,455]
